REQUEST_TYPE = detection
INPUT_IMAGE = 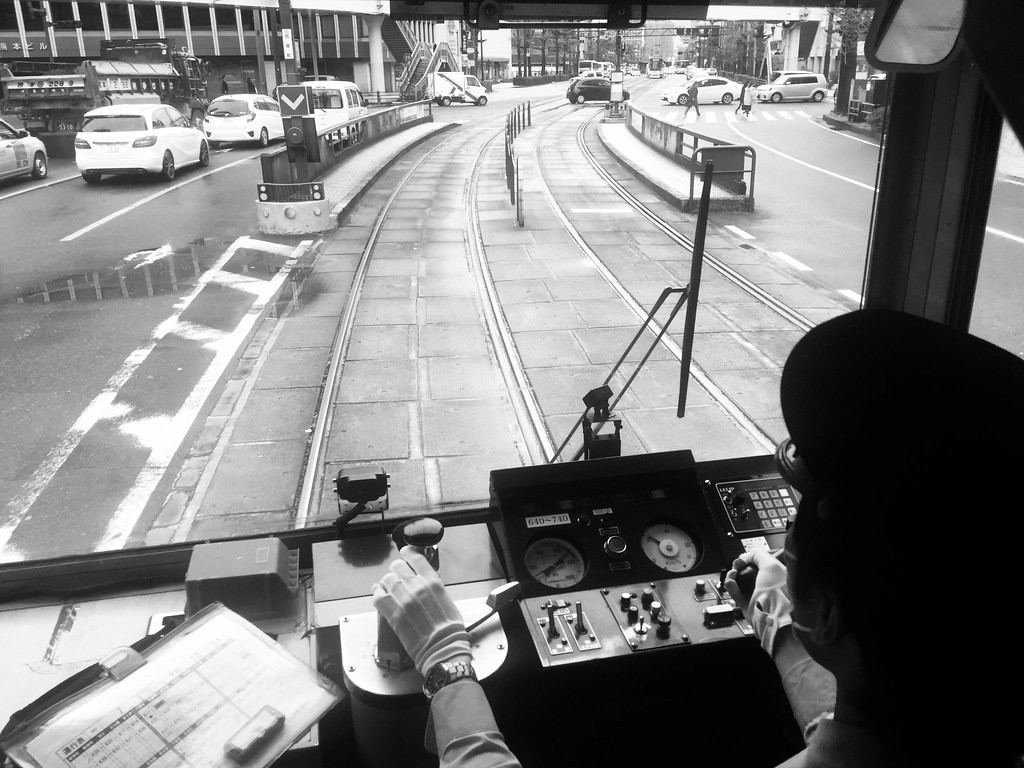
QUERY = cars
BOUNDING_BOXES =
[202,93,285,147]
[754,74,828,103]
[661,75,744,106]
[74,104,210,184]
[0,118,48,182]
[305,75,339,81]
[566,77,630,104]
[708,68,718,75]
[770,71,814,84]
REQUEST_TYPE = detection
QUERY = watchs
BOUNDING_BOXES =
[423,663,477,698]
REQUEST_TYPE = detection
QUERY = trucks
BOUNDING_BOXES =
[427,72,488,106]
[0,38,212,156]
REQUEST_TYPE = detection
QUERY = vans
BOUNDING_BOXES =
[300,80,369,147]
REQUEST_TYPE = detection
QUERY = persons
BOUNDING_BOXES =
[247,77,259,94]
[683,81,701,118]
[372,309,1024,768]
[735,81,758,117]
[221,75,228,95]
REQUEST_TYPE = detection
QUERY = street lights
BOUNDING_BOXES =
[563,51,573,74]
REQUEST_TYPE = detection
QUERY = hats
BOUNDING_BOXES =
[775,307,1023,515]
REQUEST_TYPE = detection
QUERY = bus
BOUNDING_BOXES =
[646,57,689,79]
[578,60,641,82]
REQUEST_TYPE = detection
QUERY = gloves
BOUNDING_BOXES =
[371,545,474,678]
[724,546,795,657]
